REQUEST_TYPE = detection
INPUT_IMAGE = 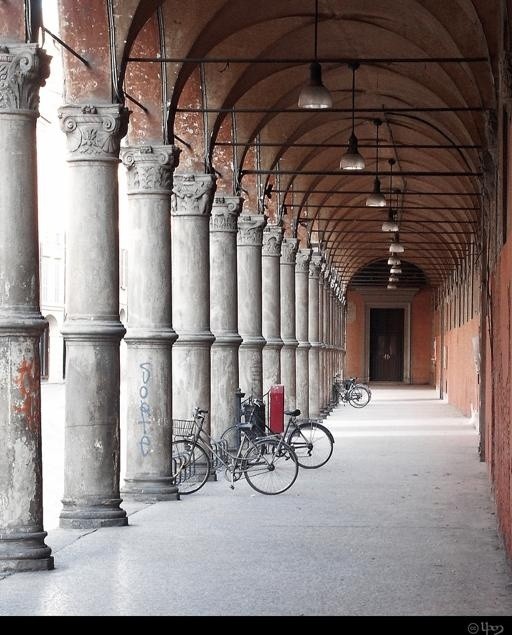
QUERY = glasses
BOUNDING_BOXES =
[298,0,404,291]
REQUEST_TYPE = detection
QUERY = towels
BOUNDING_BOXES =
[244,400,266,441]
